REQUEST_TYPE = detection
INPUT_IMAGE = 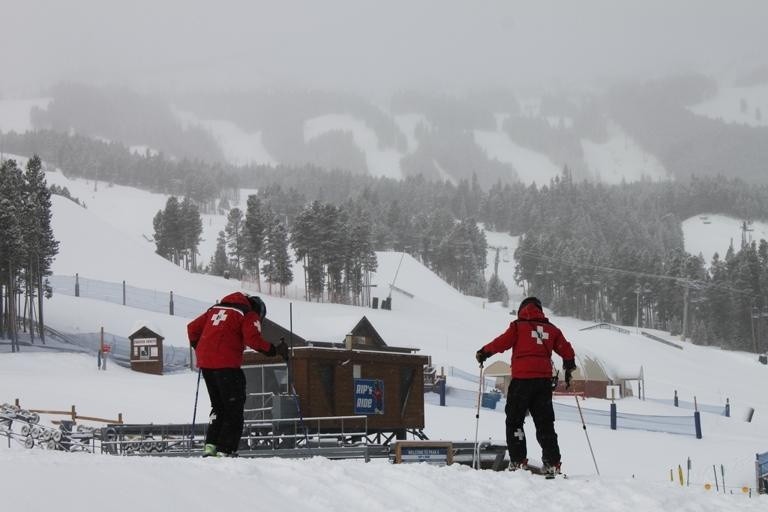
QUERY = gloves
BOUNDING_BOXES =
[565,369,575,383]
[275,341,290,356]
[476,350,488,363]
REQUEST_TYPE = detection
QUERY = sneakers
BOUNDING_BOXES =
[539,461,562,474]
[508,459,529,471]
[202,443,239,458]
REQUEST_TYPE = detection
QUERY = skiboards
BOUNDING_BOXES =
[546,471,566,478]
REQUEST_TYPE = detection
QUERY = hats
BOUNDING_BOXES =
[250,296,267,319]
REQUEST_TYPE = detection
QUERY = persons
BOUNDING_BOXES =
[475,297,577,479]
[186,290,291,460]
